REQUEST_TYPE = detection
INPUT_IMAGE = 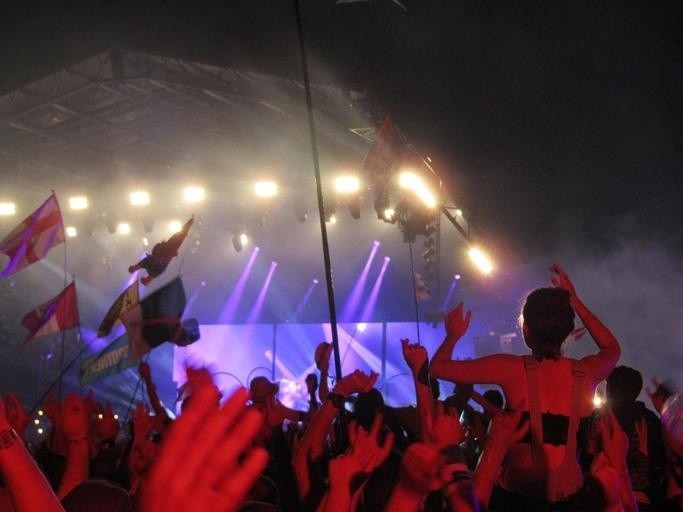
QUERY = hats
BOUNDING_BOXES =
[248,376,279,401]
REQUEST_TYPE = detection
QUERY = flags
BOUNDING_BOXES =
[0,196,65,278]
